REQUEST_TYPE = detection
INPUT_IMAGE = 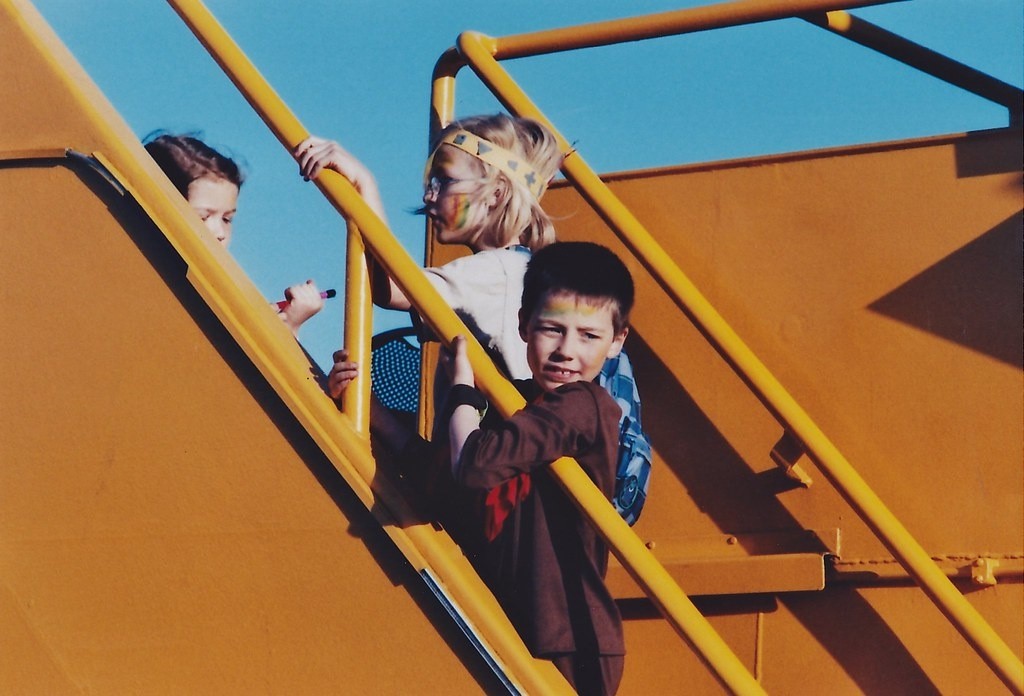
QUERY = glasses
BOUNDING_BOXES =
[424,176,494,195]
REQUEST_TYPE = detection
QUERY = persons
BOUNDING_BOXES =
[146,135,323,331]
[326,241,635,696]
[295,113,559,417]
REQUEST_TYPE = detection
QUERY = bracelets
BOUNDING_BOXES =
[448,384,488,421]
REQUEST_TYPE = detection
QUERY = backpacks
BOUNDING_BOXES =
[505,244,652,529]
